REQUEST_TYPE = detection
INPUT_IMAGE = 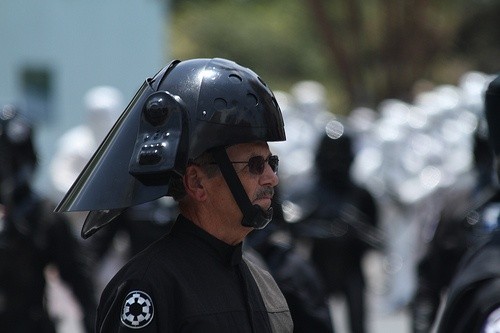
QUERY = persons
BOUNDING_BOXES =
[0,54,125,333]
[281,126,382,333]
[54,57,296,333]
[411,76,500,333]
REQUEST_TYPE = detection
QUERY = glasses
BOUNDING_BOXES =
[197,155,279,176]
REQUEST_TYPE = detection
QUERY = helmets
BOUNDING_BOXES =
[314,125,358,178]
[0,114,39,180]
[81,57,287,240]
[473,74,500,182]
